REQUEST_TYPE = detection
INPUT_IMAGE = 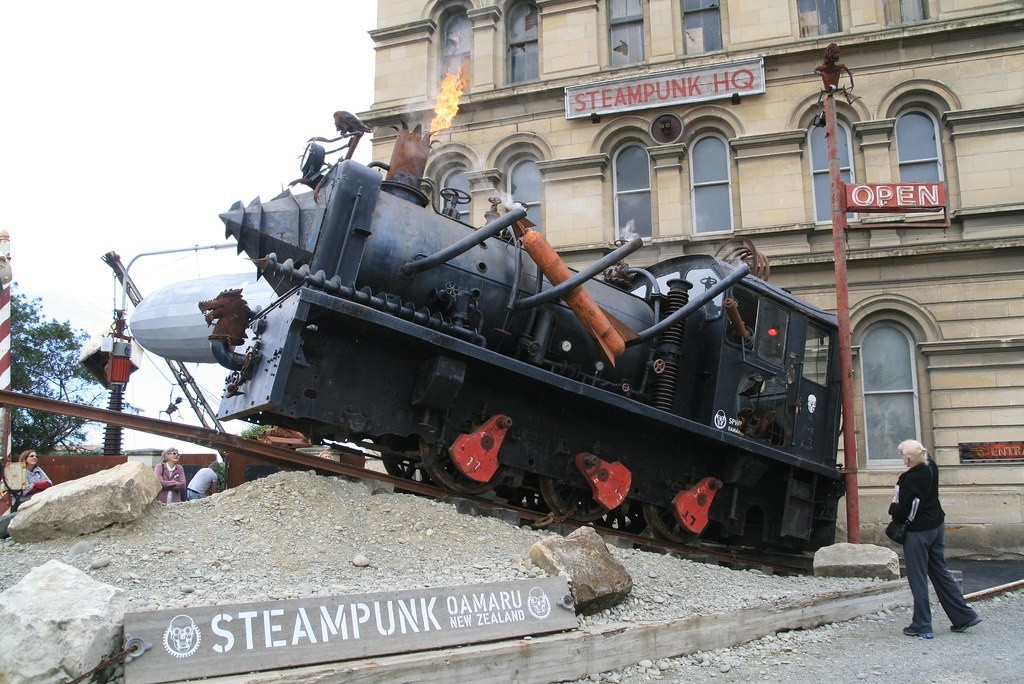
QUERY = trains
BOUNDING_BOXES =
[197,107,854,572]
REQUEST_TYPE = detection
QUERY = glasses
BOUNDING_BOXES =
[25,455,38,458]
[900,450,908,459]
[167,453,179,456]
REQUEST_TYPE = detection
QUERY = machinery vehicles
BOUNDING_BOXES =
[99,250,314,490]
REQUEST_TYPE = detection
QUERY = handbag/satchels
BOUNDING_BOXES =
[885,521,911,545]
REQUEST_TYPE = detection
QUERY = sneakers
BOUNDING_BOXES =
[950,615,982,632]
[903,627,934,638]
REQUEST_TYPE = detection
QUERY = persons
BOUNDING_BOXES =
[10,449,52,514]
[155,447,187,503]
[888,439,983,638]
[187,461,220,501]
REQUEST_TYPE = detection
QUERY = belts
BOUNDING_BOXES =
[188,488,201,494]
